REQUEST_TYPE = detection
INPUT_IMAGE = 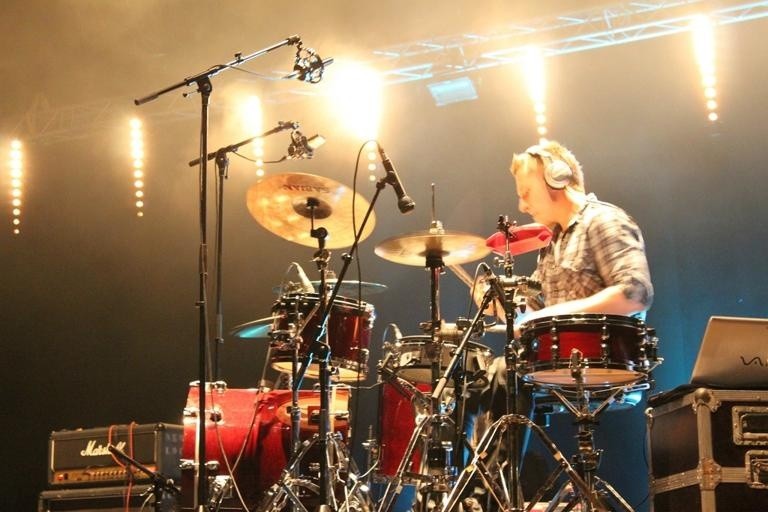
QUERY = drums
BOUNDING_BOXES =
[518,312,653,398]
[383,334,496,386]
[269,292,377,382]
[182,380,353,508]
[382,362,457,489]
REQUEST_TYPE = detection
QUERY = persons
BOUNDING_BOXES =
[468,140,661,512]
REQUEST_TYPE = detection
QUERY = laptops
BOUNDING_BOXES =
[690,314,768,388]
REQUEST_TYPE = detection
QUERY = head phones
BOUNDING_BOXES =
[524,144,573,189]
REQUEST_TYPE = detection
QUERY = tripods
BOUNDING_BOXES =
[256,228,634,512]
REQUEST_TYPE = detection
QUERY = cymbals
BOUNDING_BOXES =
[246,172,377,251]
[307,279,389,298]
[375,231,491,267]
[228,314,288,344]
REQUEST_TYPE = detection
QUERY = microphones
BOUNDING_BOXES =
[290,56,333,81]
[377,146,415,213]
[287,133,326,159]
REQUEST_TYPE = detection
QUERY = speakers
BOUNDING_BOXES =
[37,487,194,510]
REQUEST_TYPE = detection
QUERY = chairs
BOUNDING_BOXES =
[462,309,647,474]
